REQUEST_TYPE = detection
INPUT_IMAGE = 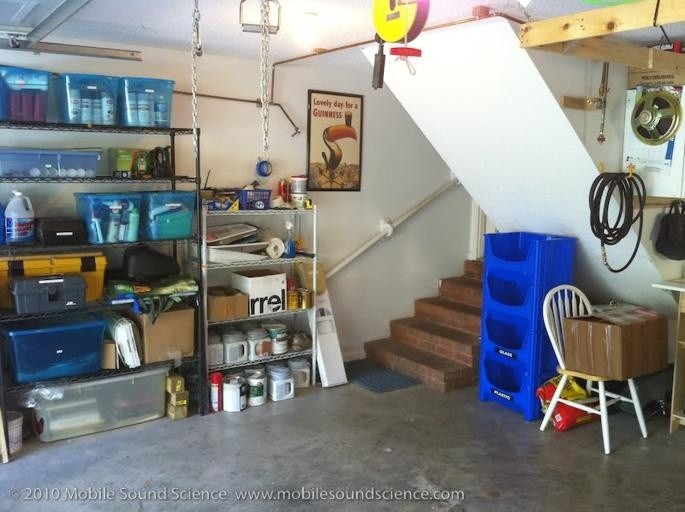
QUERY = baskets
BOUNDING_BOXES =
[224,189,272,210]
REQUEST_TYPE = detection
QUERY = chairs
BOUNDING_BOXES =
[539,284,648,454]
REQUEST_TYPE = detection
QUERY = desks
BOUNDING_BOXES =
[652,277,685,435]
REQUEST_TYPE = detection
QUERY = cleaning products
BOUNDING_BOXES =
[285,220,296,258]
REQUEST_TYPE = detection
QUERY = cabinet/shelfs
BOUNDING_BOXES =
[0,118,204,458]
[182,203,318,415]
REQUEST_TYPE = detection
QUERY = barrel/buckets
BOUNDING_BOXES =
[209,322,289,366]
[7,411,25,454]
[220,357,314,413]
[5,189,37,244]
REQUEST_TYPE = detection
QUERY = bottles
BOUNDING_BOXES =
[67,88,168,126]
[278,176,287,203]
[9,91,47,122]
[107,208,140,243]
[210,371,223,411]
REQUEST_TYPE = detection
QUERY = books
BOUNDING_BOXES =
[44,388,161,433]
[95,309,142,370]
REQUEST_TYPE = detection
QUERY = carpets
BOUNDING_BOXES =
[343,358,422,393]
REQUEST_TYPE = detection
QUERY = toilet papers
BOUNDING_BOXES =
[263,227,286,259]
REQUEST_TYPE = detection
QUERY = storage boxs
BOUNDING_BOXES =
[206,285,249,323]
[52,73,120,126]
[117,77,175,128]
[128,300,195,365]
[74,193,143,245]
[231,269,288,318]
[0,250,108,309]
[0,64,51,124]
[0,313,107,385]
[131,190,196,241]
[8,272,88,316]
[31,366,172,442]
[562,306,668,382]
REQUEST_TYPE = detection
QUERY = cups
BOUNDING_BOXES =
[345,111,352,128]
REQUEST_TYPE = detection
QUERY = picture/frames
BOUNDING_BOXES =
[306,89,363,191]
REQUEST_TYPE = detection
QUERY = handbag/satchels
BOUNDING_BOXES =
[656,213,685,260]
